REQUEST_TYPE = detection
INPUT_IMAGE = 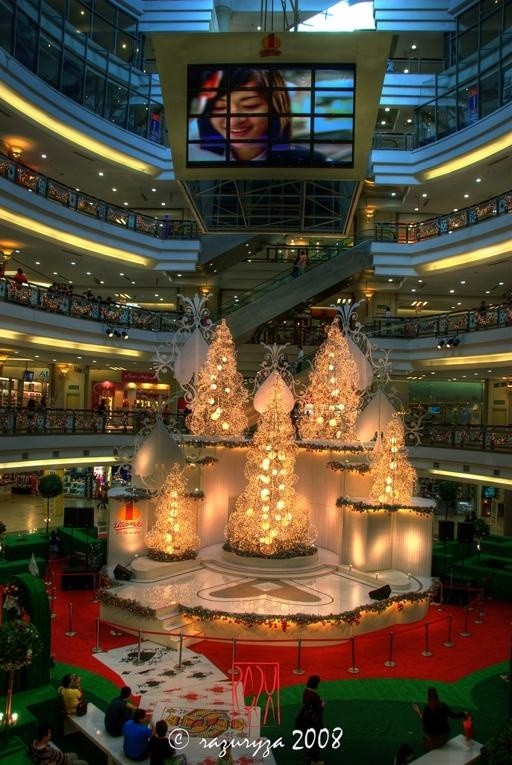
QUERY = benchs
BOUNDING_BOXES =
[408,734,483,765]
[66,703,150,765]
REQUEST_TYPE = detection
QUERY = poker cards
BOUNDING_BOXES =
[93,640,277,763]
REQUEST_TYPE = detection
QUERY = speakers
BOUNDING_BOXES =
[439,520,453,541]
[457,521,474,544]
[63,507,94,529]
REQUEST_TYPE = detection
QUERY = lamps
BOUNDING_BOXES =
[435,329,460,350]
[106,323,130,340]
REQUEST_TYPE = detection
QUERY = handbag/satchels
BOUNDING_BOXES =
[77,702,87,716]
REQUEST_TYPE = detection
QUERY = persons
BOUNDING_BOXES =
[294,249,307,278]
[24,720,88,765]
[411,686,471,752]
[476,299,493,332]
[384,306,395,332]
[189,66,234,161]
[57,673,83,715]
[200,66,327,163]
[441,315,452,334]
[104,685,189,765]
[295,346,304,373]
[26,396,191,435]
[0,246,116,322]
[296,674,328,765]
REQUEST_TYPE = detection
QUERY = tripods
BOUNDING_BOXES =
[452,544,475,589]
